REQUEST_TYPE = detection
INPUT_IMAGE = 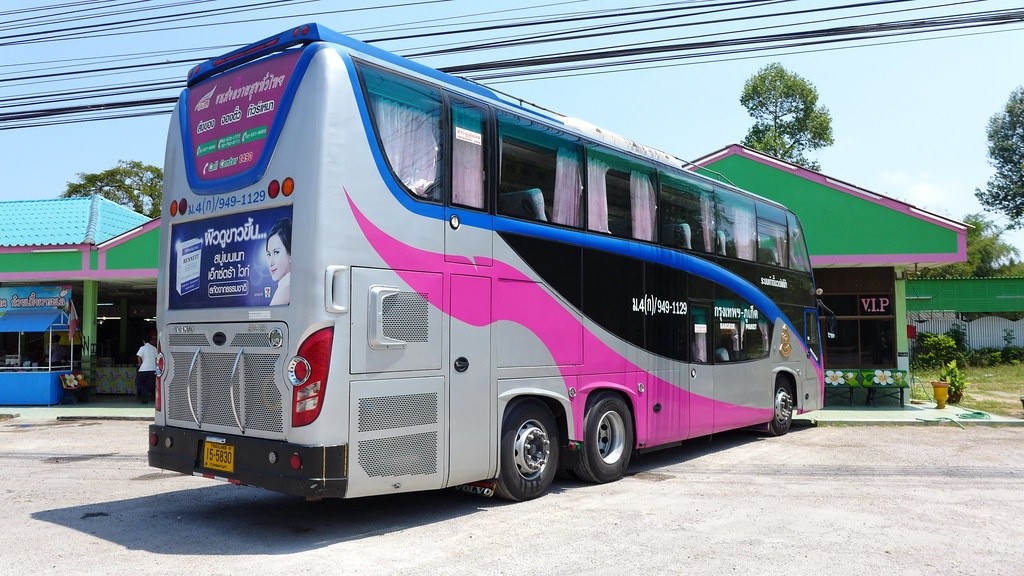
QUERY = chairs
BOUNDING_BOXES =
[698,228,727,257]
[658,223,694,251]
[499,188,549,223]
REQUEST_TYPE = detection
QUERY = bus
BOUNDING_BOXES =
[145,21,840,507]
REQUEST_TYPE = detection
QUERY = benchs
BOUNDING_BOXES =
[59,373,98,405]
[825,368,909,409]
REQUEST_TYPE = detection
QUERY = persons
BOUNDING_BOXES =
[266,217,292,306]
[136,335,157,404]
[51,335,66,371]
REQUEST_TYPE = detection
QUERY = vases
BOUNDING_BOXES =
[930,381,950,407]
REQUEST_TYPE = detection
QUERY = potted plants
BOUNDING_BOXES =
[941,359,967,404]
